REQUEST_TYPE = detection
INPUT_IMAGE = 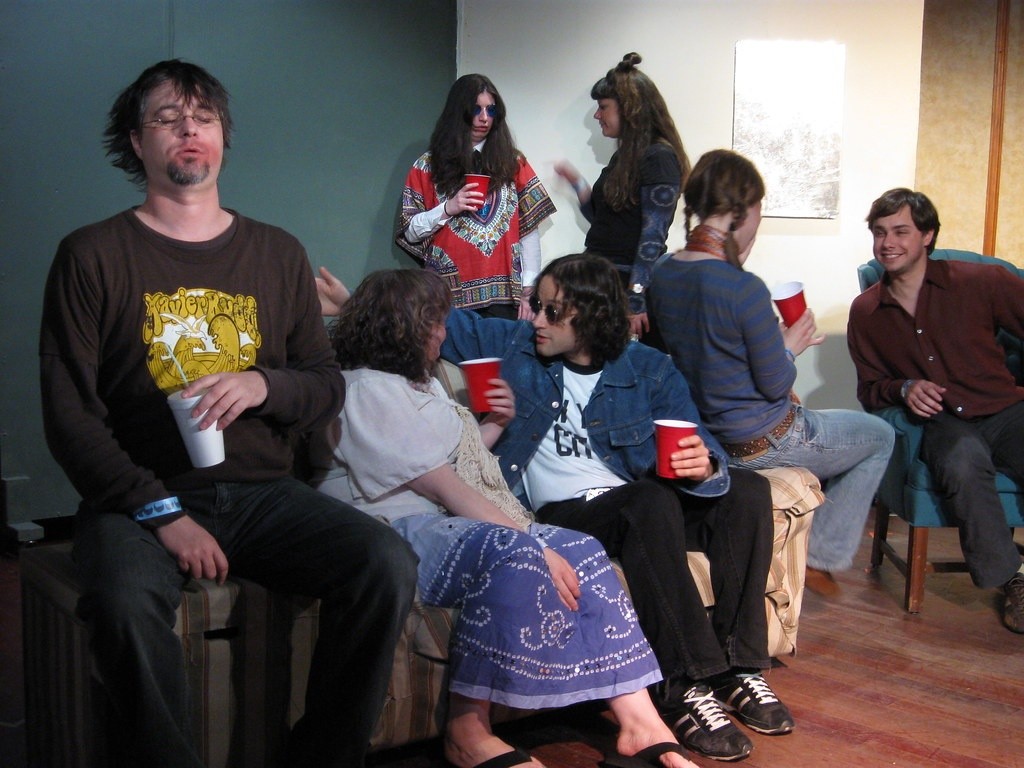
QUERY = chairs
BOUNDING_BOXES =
[858,249,1024,614]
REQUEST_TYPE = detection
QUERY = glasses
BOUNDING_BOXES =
[529,294,578,323]
[474,105,496,116]
[140,110,223,129]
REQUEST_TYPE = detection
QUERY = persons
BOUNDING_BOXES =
[38,59,419,768]
[847,189,1024,634]
[319,271,707,768]
[640,149,896,602]
[316,255,795,761]
[392,74,557,321]
[553,52,694,318]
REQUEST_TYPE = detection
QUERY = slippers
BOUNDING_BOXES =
[604,741,691,768]
[473,746,534,768]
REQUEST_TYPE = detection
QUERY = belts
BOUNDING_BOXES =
[720,407,795,458]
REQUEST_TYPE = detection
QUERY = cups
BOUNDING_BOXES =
[653,418,699,479]
[458,356,504,414]
[168,385,225,469]
[770,281,807,328]
[464,173,491,209]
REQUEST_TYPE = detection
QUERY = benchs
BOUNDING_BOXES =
[369,357,826,756]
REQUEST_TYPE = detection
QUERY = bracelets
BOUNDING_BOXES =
[535,538,548,549]
[901,380,912,402]
[708,450,719,475]
[785,349,795,362]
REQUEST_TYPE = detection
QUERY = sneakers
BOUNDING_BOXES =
[658,681,753,760]
[1002,572,1024,633]
[708,667,794,734]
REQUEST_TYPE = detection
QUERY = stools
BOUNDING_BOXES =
[22,542,321,768]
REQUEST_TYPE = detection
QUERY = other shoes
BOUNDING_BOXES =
[803,565,837,599]
